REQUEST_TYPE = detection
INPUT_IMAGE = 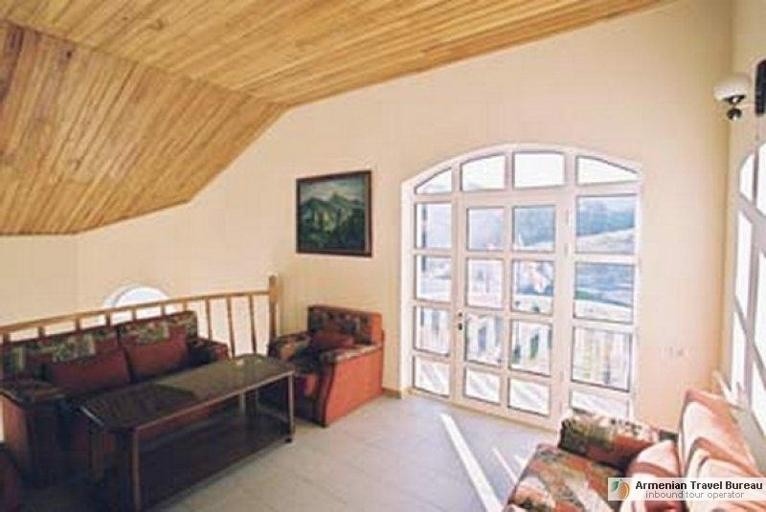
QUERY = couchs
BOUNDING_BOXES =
[2,308,236,491]
[499,387,765,511]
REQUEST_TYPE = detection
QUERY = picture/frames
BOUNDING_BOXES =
[295,169,374,257]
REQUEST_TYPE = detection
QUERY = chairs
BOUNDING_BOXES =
[259,306,385,428]
[1,449,24,512]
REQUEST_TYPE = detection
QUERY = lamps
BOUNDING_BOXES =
[711,58,766,121]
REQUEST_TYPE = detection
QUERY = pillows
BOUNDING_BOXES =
[43,350,131,405]
[616,439,684,512]
[311,329,353,363]
[129,342,187,383]
